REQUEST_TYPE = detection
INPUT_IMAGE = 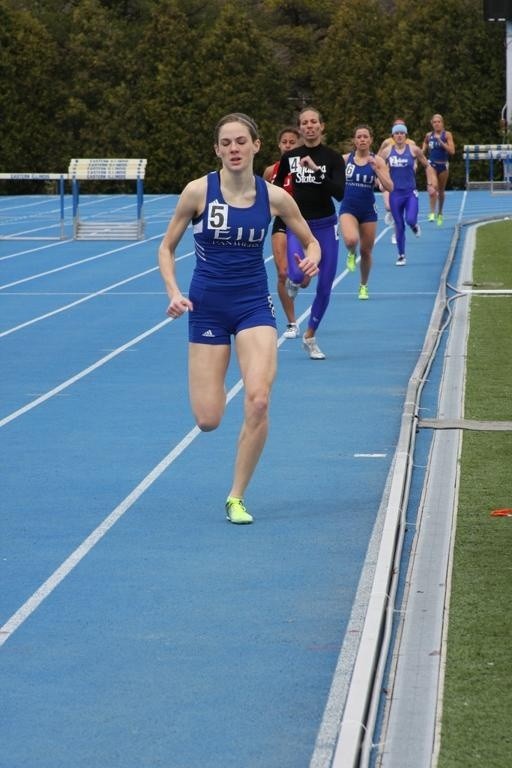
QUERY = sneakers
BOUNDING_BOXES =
[284,323,301,339]
[384,211,420,244]
[357,283,369,298]
[302,332,326,361]
[395,254,408,267]
[428,212,443,226]
[224,498,254,524]
[285,279,301,298]
[347,251,356,271]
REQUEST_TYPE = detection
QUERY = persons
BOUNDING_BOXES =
[158,113,322,525]
[262,127,310,338]
[273,106,345,359]
[378,113,455,266]
[339,124,394,299]
